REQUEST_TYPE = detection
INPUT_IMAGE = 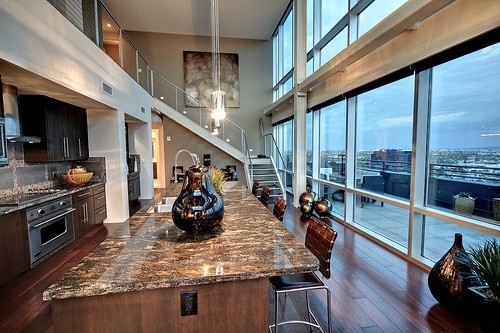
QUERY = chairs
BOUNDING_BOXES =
[226,164,238,181]
[361,174,385,208]
[170,165,184,183]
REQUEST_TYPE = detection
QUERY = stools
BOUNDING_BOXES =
[252,180,259,196]
[261,186,271,207]
[273,197,287,221]
[269,216,337,333]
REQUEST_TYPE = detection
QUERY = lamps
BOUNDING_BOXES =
[208,0,228,133]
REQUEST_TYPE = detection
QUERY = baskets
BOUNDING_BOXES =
[61,171,94,185]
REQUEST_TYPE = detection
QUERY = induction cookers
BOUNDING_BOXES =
[0,190,73,224]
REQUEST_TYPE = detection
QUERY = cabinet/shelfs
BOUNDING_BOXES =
[93,181,106,226]
[75,105,89,161]
[18,94,76,162]
[0,212,31,285]
[71,188,94,235]
[128,171,141,201]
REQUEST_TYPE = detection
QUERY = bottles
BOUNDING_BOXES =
[428,233,479,310]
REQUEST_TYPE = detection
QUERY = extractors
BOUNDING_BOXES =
[2,83,41,143]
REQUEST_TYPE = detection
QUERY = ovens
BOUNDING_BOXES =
[26,207,77,269]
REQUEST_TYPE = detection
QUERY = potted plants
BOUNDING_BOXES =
[451,192,477,213]
[451,239,500,333]
